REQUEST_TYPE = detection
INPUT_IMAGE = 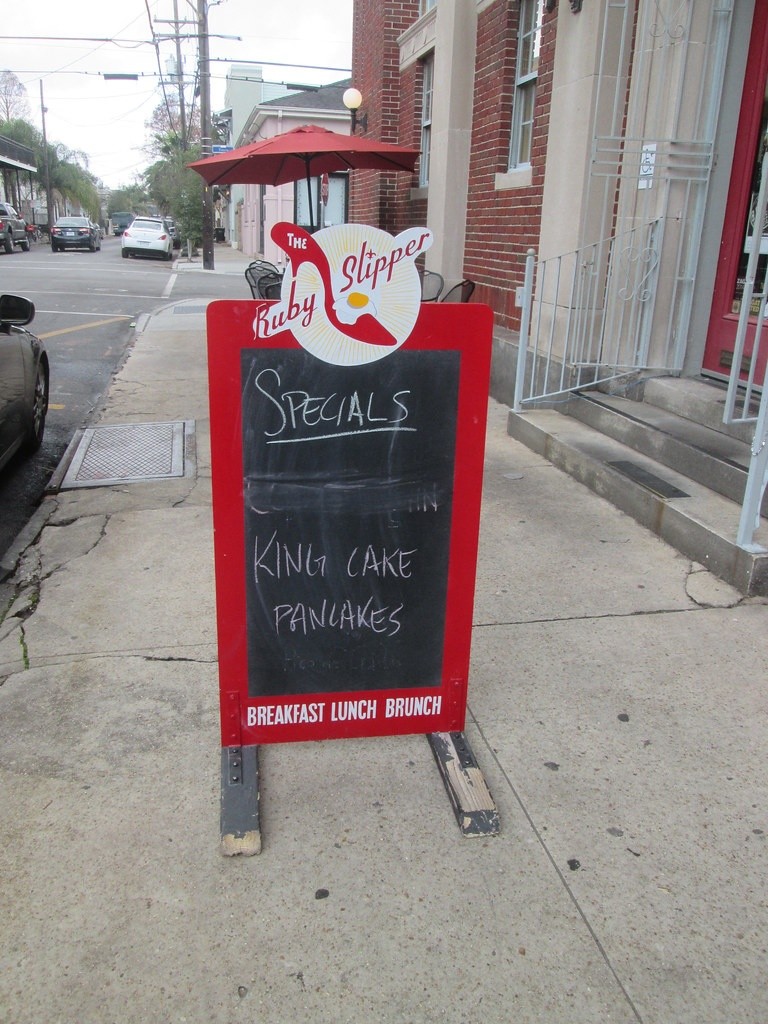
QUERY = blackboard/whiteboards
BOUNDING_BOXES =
[206,302,496,745]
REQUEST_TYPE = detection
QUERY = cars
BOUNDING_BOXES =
[0,294,50,473]
[50,216,101,252]
[153,215,181,249]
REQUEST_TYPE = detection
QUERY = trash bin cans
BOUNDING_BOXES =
[215,226,226,242]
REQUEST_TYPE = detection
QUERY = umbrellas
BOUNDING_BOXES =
[184,123,424,233]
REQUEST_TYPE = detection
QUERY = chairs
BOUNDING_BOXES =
[244,259,476,303]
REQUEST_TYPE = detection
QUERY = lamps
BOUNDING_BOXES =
[342,88,368,133]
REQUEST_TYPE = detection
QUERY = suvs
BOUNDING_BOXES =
[0,202,30,253]
[121,214,173,260]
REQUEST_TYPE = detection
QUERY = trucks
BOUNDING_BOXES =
[112,212,135,237]
